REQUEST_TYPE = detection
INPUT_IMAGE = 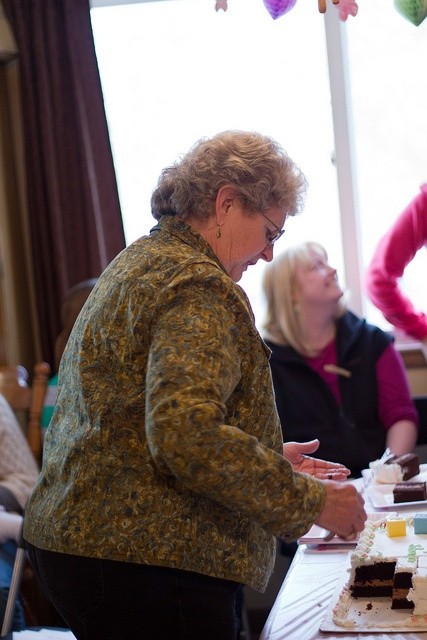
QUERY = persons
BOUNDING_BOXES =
[365,182,426,348]
[0,391,40,632]
[261,241,419,479]
[22,129,369,640]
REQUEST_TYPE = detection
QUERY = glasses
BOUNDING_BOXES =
[255,206,285,247]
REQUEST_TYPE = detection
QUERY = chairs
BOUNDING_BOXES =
[0,362,51,458]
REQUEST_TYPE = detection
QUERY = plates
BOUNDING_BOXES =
[363,483,427,507]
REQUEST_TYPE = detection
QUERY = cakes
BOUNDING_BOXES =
[380,482,424,502]
[347,516,427,617]
[369,451,419,481]
